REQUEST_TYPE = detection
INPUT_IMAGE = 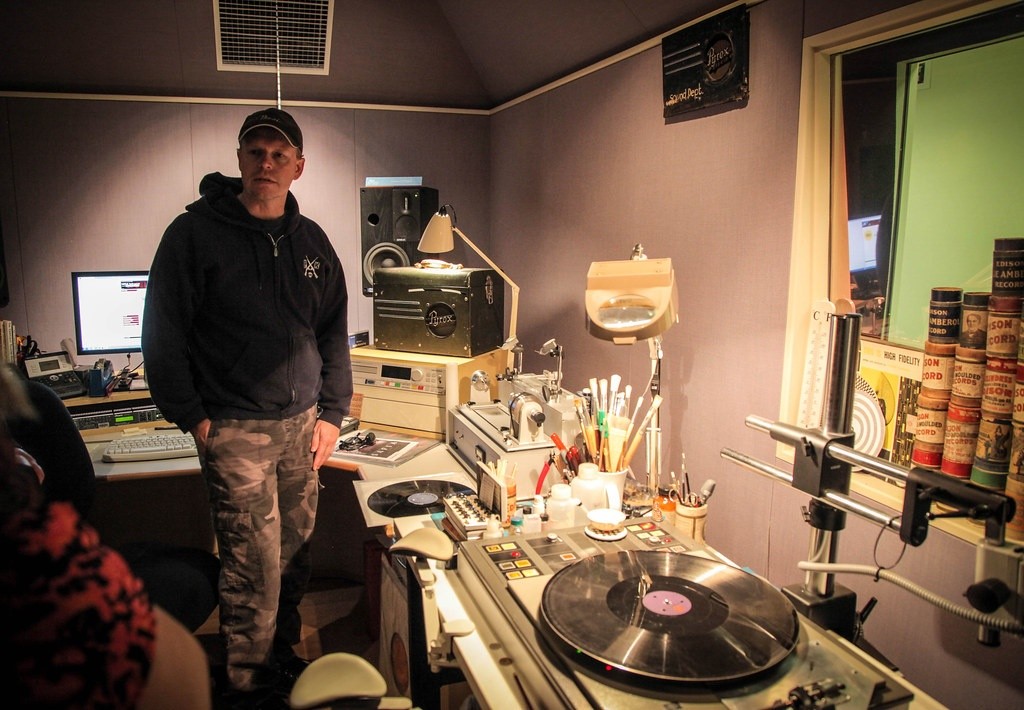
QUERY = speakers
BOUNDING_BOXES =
[359,187,440,298]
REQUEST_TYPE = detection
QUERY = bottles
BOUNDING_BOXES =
[484,463,607,539]
[653,486,678,527]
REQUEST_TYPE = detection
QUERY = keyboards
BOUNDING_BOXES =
[101,434,197,460]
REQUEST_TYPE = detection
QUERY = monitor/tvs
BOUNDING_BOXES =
[70,269,151,392]
[848,213,881,300]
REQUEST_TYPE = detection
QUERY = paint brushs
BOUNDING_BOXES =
[669,450,716,505]
[573,375,663,473]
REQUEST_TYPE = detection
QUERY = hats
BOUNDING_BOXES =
[237,107,303,151]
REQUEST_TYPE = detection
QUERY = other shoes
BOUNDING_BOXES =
[275,657,315,705]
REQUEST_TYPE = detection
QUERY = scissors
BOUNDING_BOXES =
[668,489,699,508]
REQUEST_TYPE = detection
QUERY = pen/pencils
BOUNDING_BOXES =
[154,427,178,429]
[551,434,580,475]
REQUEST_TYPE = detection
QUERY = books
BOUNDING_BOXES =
[0,320,18,367]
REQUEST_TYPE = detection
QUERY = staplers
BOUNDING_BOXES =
[118,368,132,388]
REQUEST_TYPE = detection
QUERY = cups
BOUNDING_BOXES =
[599,468,630,514]
[676,498,707,542]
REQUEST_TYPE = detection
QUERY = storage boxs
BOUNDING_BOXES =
[372,266,505,357]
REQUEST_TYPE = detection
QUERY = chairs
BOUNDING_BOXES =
[10,378,412,710]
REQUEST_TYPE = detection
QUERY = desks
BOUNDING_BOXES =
[62,390,359,481]
[358,445,523,710]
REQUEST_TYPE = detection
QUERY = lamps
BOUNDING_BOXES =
[416,204,521,373]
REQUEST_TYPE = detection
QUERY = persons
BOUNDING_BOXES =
[0,370,210,710]
[141,107,354,710]
